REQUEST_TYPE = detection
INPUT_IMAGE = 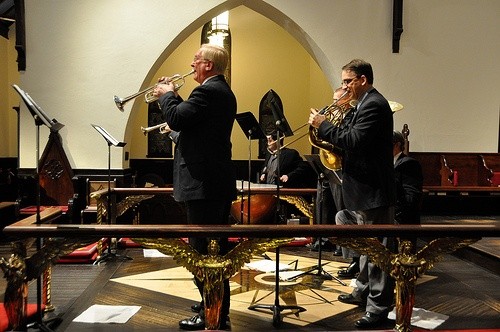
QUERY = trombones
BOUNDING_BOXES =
[266,99,353,155]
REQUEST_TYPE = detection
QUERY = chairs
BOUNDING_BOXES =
[67,174,129,225]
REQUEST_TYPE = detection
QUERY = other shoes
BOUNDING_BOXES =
[337,262,360,278]
[333,250,342,256]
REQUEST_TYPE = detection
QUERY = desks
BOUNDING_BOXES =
[239,188,320,251]
[20,204,71,214]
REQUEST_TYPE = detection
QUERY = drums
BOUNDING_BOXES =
[230,193,275,226]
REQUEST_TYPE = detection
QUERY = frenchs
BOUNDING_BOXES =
[310,91,349,172]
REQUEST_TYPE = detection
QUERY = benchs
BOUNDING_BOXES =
[412,153,500,193]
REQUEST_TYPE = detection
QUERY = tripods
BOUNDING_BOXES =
[248,103,307,330]
[287,154,347,286]
[12,85,65,332]
[92,124,133,265]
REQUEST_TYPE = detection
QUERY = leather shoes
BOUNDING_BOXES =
[354,317,381,329]
[179,314,227,330]
[338,294,363,305]
[191,304,203,311]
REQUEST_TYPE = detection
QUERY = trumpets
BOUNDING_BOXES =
[113,69,196,112]
[141,122,170,136]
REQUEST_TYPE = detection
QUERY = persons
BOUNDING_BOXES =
[307,87,354,256]
[309,58,398,328]
[334,129,424,277]
[152,44,238,330]
[260,129,308,189]
